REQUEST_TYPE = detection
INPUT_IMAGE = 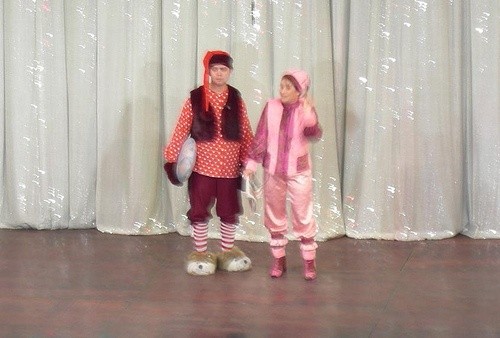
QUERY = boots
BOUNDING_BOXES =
[303,250,317,280]
[271,246,287,277]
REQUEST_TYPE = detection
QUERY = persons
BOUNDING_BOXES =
[163,50,255,277]
[243,69,322,280]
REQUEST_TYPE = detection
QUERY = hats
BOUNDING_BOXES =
[283,70,311,98]
[203,51,233,112]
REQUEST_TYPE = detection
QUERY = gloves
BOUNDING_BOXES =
[164,162,183,186]
[238,175,246,192]
